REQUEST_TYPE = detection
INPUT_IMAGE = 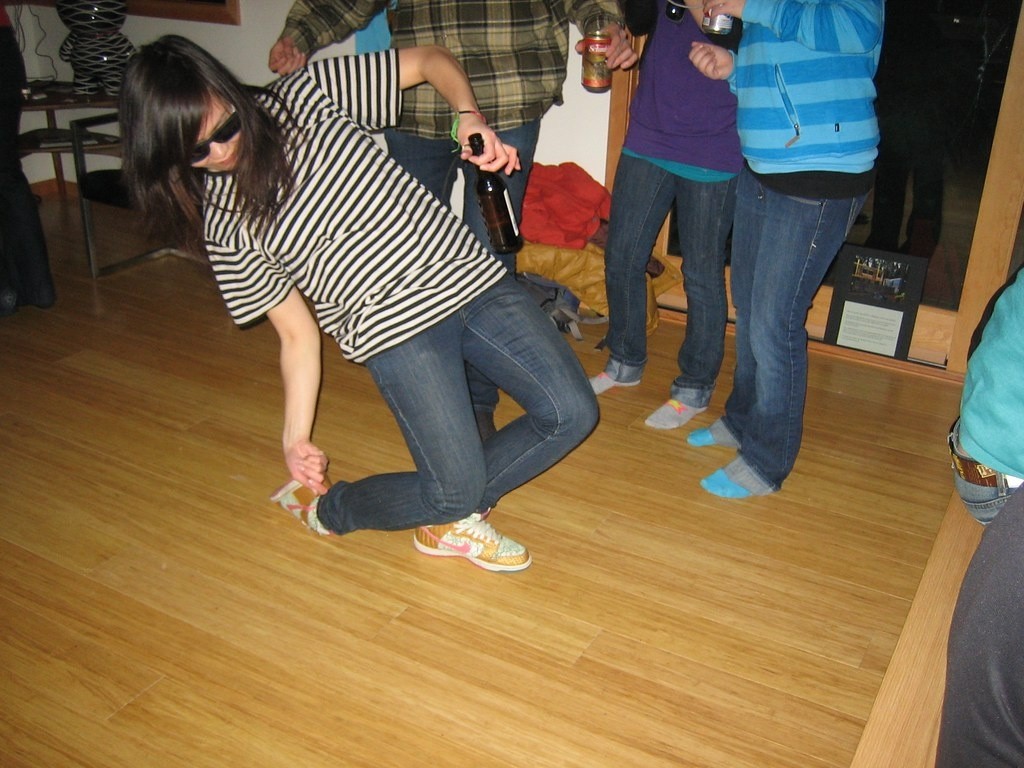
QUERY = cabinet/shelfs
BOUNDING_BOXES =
[15,79,213,282]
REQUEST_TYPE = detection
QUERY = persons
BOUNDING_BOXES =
[588,0,743,428]
[118,35,601,572]
[936,482,1023,768]
[0,6,55,315]
[948,267,1023,526]
[863,0,1015,303]
[269,0,639,441]
[686,0,885,499]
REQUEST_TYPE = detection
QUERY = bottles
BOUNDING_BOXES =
[468,133,524,254]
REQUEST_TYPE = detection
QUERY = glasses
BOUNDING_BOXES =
[191,108,242,162]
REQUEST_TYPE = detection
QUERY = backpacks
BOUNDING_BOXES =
[513,271,608,341]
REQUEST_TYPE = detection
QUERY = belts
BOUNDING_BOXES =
[947,414,1009,487]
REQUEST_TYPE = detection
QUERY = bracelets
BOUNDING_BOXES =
[450,110,487,152]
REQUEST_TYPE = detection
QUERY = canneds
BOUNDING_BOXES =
[582,33,612,92]
[702,4,734,35]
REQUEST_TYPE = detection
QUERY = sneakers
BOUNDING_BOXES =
[269,468,335,538]
[411,515,534,573]
